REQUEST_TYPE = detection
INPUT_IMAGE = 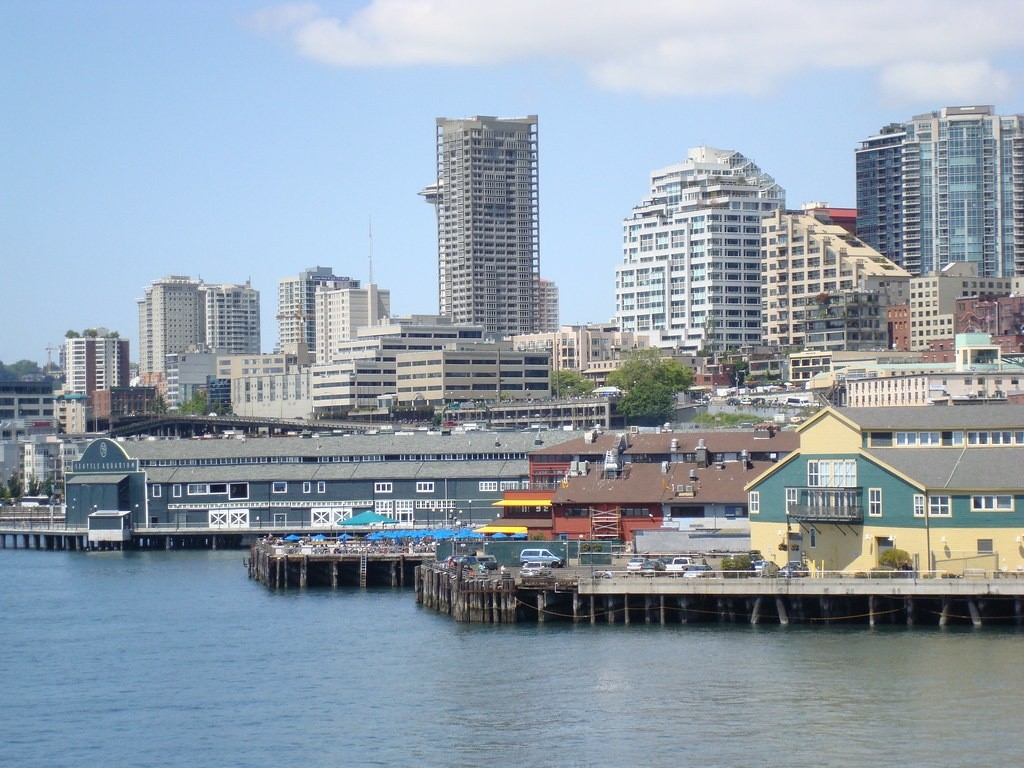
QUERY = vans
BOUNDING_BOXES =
[519,547,566,569]
[444,555,498,572]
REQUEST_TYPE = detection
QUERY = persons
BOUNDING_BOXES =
[501,564,505,574]
[448,558,486,579]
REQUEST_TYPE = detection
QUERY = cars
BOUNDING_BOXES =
[519,561,554,580]
[726,396,765,407]
[748,549,807,578]
[627,556,716,579]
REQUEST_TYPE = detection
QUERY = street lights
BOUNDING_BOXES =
[440,508,444,529]
[469,499,472,526]
[432,507,436,529]
[454,516,458,527]
[459,510,463,528]
[450,508,454,527]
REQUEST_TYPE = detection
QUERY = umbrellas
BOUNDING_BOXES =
[336,510,400,525]
[285,529,529,545]
